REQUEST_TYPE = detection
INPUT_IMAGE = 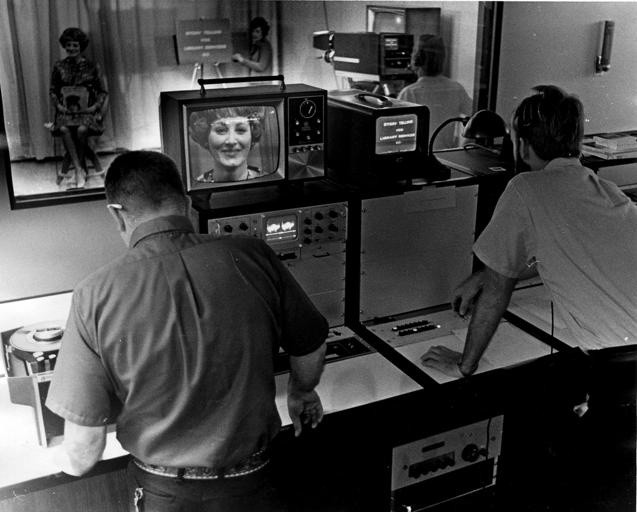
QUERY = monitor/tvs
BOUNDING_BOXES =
[159,83,329,196]
[366,5,441,71]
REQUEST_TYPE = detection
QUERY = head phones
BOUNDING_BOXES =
[414,48,425,68]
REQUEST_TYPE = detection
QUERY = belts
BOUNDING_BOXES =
[133,444,274,481]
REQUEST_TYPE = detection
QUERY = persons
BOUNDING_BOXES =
[419,84,635,512]
[231,15,273,86]
[43,148,330,512]
[394,33,472,150]
[186,107,269,183]
[48,25,110,192]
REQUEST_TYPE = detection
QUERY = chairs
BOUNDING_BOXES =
[50,75,106,185]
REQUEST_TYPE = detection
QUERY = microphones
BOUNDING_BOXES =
[463,142,501,155]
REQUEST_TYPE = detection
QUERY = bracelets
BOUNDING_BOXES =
[456,360,478,377]
